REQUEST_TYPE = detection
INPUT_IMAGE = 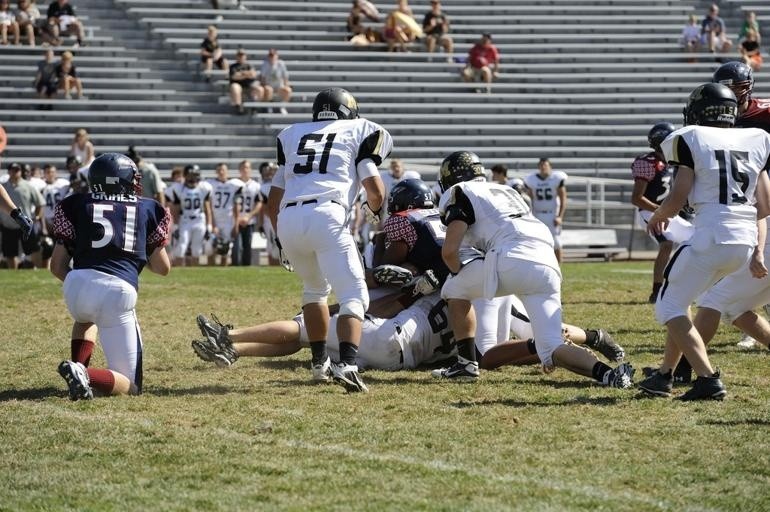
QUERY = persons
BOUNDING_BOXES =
[43,165,71,269]
[737,11,761,44]
[348,5,381,41]
[259,48,292,115]
[399,0,422,42]
[347,190,379,245]
[267,88,393,391]
[633,83,770,402]
[191,287,472,372]
[381,158,421,211]
[32,49,61,110]
[356,1,380,21]
[47,0,84,48]
[170,164,213,268]
[431,150,635,388]
[71,128,97,167]
[201,26,229,81]
[44,168,76,269]
[165,167,185,266]
[462,33,500,94]
[678,14,703,62]
[205,162,247,266]
[64,155,88,199]
[424,0,454,63]
[51,152,170,400]
[0,162,47,269]
[54,51,89,99]
[711,62,769,348]
[699,5,732,64]
[124,144,166,205]
[631,122,692,304]
[383,12,426,52]
[32,166,41,178]
[385,178,625,370]
[22,164,46,269]
[0,0,19,45]
[523,158,568,265]
[18,0,64,49]
[259,161,296,272]
[231,161,263,265]
[737,22,763,71]
[228,48,264,115]
[491,165,522,200]
[0,182,34,241]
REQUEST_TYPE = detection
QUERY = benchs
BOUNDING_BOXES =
[75,13,90,21]
[138,16,708,32]
[0,158,255,171]
[387,133,654,146]
[569,169,634,178]
[212,83,770,97]
[567,189,634,206]
[737,6,770,13]
[252,113,690,124]
[241,100,691,114]
[217,90,693,107]
[1,45,127,58]
[190,59,728,74]
[163,36,690,55]
[123,7,710,25]
[0,32,113,47]
[0,86,177,101]
[4,135,228,147]
[112,0,696,16]
[0,122,219,135]
[205,64,769,84]
[0,75,164,89]
[387,146,657,158]
[0,4,77,18]
[4,146,241,159]
[150,24,740,42]
[267,120,688,137]
[753,16,770,22]
[0,98,190,112]
[0,56,140,67]
[81,26,102,36]
[382,157,635,168]
[0,65,153,78]
[177,46,766,64]
[0,110,204,122]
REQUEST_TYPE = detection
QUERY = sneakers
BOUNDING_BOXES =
[431,354,480,380]
[330,360,369,393]
[597,362,637,388]
[561,328,600,362]
[638,368,675,397]
[191,339,240,367]
[310,355,337,383]
[400,268,440,300]
[196,312,233,352]
[57,359,94,401]
[587,328,625,362]
[372,264,413,286]
[672,367,728,402]
[737,336,769,351]
[642,366,692,384]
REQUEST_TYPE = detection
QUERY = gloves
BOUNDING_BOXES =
[360,201,382,225]
[9,207,34,242]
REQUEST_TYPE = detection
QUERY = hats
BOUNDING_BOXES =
[7,163,22,171]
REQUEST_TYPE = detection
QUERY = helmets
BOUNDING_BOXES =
[711,61,755,106]
[438,151,485,194]
[212,237,230,255]
[274,237,296,273]
[647,122,676,148]
[182,164,201,183]
[259,161,273,173]
[386,178,434,215]
[682,82,738,127]
[311,87,360,122]
[65,154,82,168]
[87,152,140,194]
